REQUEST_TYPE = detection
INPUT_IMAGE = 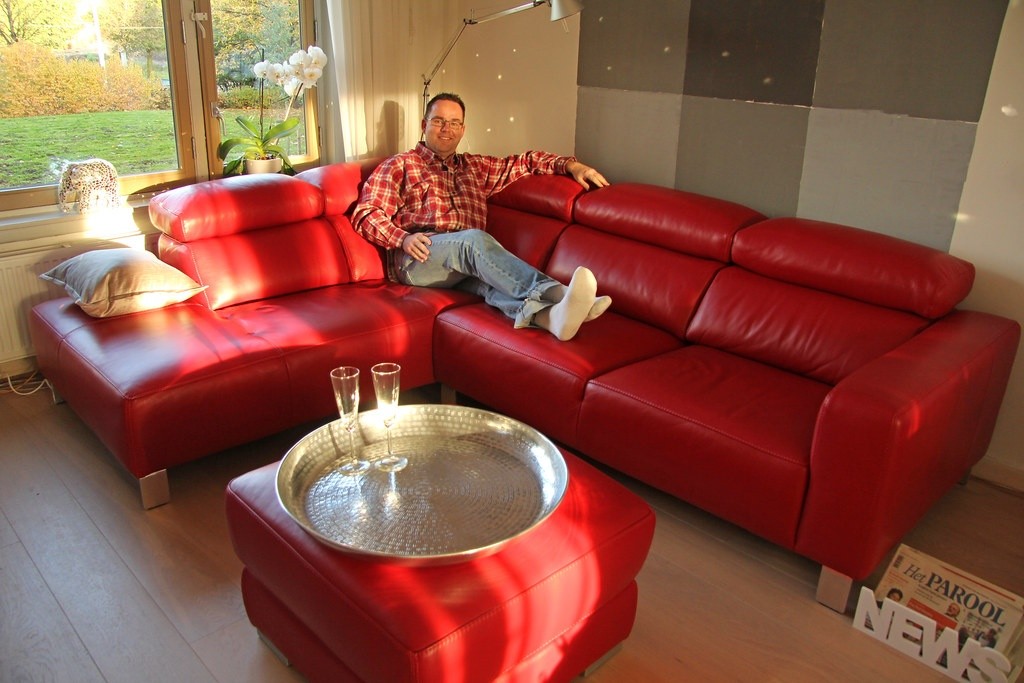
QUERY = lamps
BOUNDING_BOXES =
[420,0,587,141]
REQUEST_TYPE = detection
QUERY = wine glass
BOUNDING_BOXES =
[329,365,371,475]
[371,362,409,472]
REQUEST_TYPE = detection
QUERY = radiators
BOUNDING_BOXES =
[0,225,162,363]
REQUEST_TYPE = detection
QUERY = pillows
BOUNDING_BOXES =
[38,248,208,317]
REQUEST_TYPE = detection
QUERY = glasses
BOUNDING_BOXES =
[424,116,465,129]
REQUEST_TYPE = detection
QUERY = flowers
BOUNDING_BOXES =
[218,45,328,176]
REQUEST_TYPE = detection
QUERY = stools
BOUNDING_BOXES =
[224,444,656,683]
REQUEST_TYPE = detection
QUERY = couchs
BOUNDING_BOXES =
[28,152,1022,614]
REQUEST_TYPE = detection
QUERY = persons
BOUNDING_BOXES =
[348,91,613,341]
[875,587,903,610]
[943,602,961,622]
[975,627,998,649]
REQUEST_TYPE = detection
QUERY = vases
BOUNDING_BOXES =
[245,158,282,174]
